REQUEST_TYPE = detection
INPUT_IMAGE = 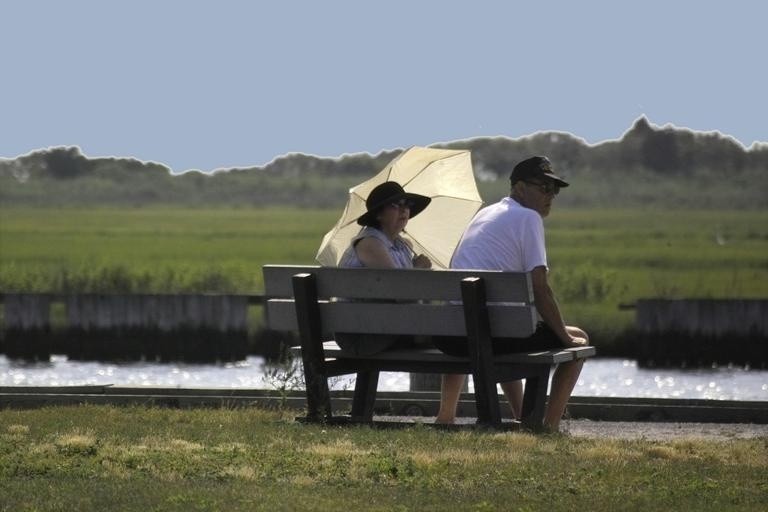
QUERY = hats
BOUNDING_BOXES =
[359,183,431,226]
[511,157,569,185]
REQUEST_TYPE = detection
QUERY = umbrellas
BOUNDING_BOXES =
[315,145,484,270]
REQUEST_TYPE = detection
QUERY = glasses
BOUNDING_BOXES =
[392,200,415,209]
[530,183,560,194]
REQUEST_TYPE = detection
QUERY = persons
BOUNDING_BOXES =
[334,182,467,426]
[448,157,589,432]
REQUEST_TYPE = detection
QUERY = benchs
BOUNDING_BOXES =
[257,257,597,431]
